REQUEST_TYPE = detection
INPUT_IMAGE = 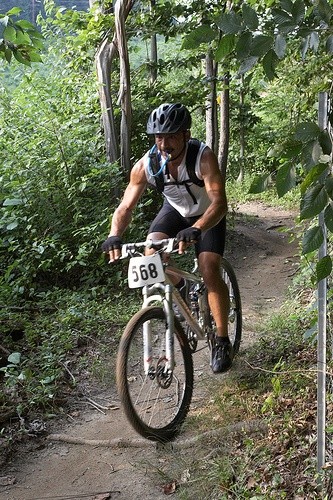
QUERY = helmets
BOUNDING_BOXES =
[146,102,192,136]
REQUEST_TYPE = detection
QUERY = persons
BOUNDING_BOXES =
[99,102,234,375]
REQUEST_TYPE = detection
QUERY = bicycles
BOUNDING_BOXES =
[104,236,243,442]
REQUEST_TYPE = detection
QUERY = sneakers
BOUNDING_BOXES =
[172,281,191,323]
[212,338,235,375]
